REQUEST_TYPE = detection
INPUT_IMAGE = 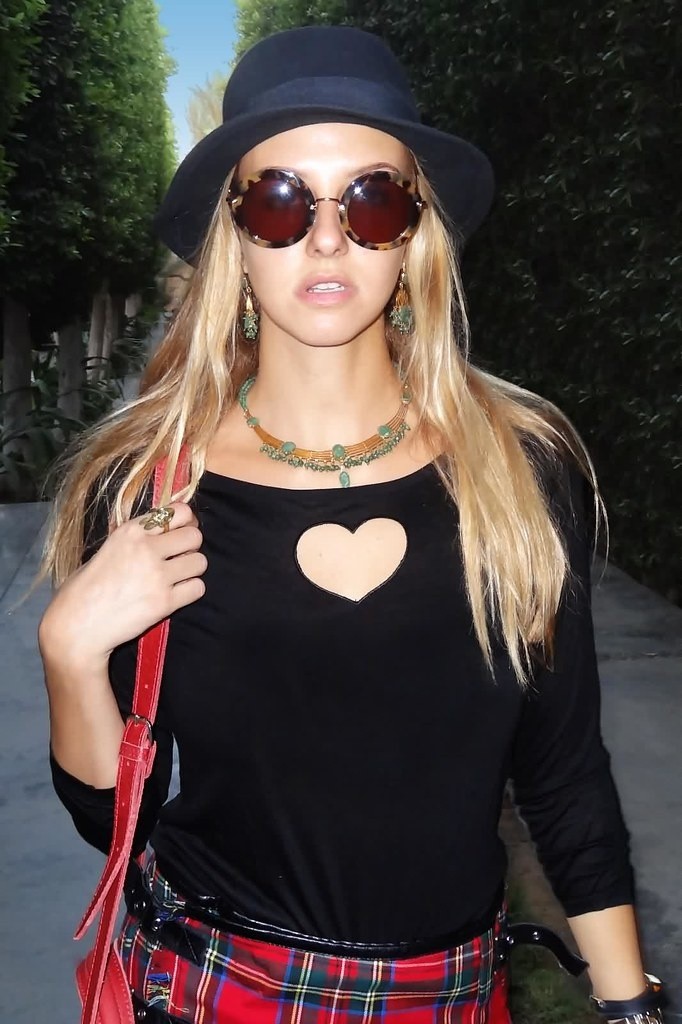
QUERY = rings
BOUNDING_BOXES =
[142,505,175,534]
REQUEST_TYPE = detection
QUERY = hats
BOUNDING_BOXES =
[156,26,497,268]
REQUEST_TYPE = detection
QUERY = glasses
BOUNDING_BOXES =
[225,164,427,250]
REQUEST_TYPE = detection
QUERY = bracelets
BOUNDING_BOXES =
[590,972,665,1019]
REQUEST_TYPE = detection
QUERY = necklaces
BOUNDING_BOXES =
[238,374,414,485]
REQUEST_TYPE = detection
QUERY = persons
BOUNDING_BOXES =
[30,25,668,1023]
